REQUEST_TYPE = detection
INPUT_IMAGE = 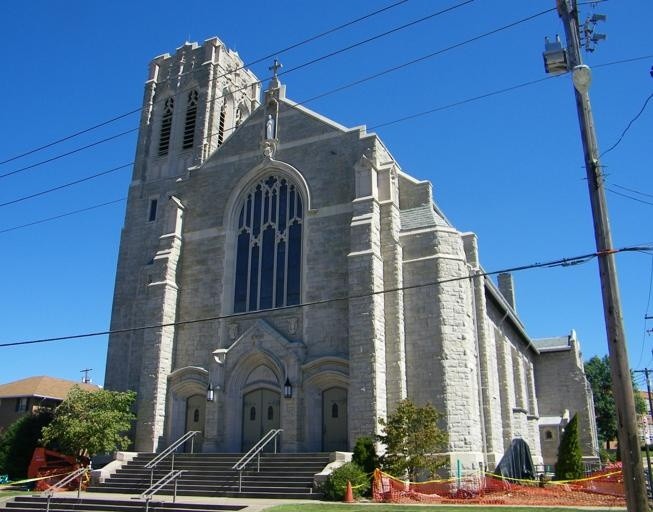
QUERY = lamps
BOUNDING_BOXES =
[280,375,292,399]
[204,382,219,401]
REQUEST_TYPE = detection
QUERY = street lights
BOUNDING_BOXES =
[570,64,649,512]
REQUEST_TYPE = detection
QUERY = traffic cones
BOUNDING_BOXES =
[342,480,357,502]
